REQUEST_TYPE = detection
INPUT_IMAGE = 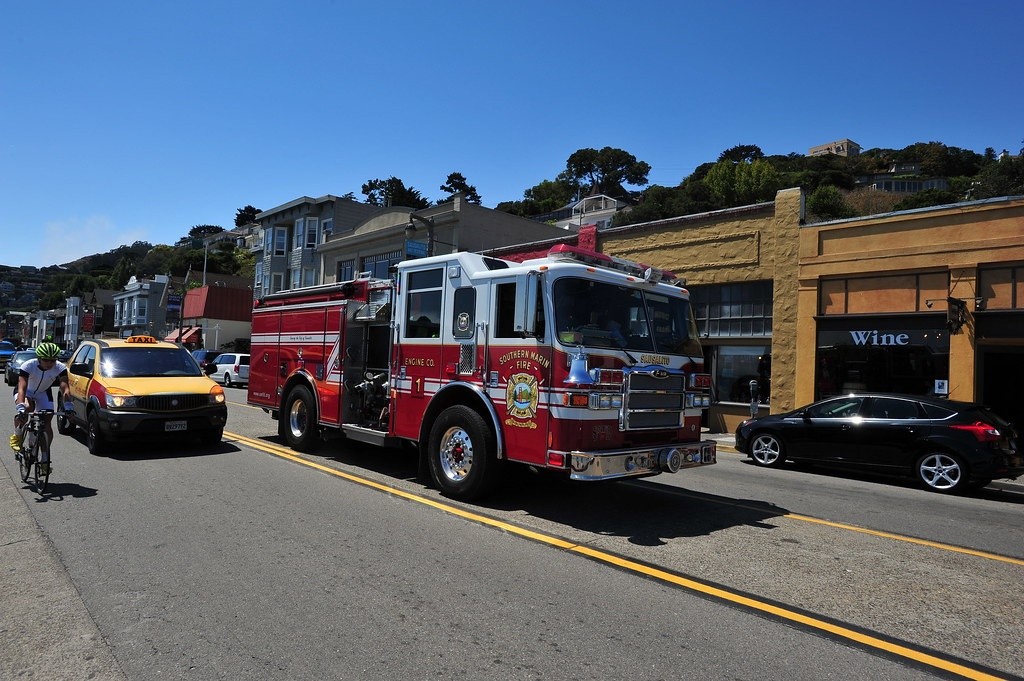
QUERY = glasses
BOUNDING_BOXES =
[41,359,56,363]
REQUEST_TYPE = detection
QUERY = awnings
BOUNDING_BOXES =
[164,326,202,343]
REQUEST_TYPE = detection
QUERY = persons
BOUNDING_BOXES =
[201,359,210,378]
[9,342,71,475]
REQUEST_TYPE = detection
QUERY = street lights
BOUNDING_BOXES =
[81,302,104,339]
[23,318,36,346]
[168,278,200,343]
[403,212,457,257]
[47,311,60,344]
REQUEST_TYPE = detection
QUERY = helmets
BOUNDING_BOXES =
[35,343,60,359]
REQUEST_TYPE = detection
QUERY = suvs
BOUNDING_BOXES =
[56,334,228,457]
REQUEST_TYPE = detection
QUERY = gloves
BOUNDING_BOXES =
[15,403,25,414]
[64,402,75,411]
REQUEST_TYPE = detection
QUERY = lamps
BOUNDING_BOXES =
[925,298,946,308]
[976,296,984,308]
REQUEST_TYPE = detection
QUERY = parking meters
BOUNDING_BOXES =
[748,379,759,419]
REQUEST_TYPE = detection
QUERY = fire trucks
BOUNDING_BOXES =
[246,242,719,502]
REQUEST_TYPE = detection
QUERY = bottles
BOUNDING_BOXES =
[31,433,38,448]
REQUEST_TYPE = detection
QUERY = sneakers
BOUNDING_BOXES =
[9,434,22,451]
[41,464,51,474]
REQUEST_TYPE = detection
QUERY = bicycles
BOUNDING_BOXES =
[13,409,76,496]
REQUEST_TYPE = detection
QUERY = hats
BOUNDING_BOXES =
[750,380,757,385]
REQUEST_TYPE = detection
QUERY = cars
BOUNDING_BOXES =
[0,339,75,388]
[732,390,1024,493]
[201,352,251,388]
[184,348,225,371]
